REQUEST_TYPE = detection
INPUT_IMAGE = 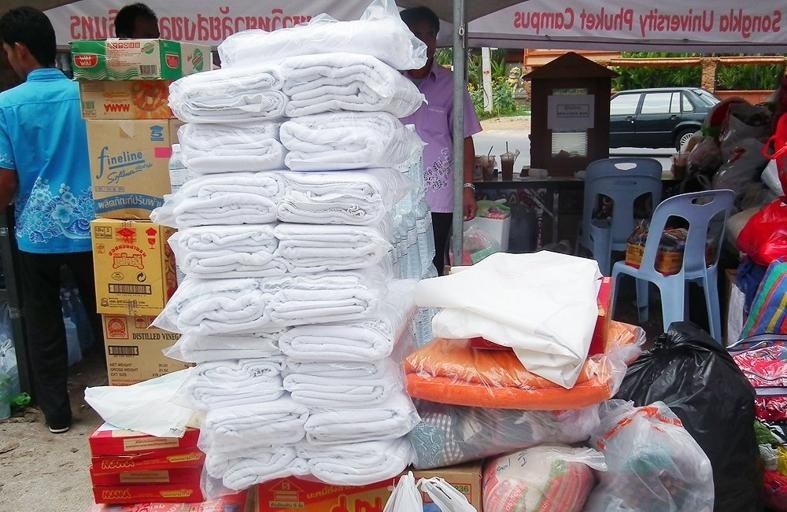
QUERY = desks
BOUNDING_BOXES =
[474,168,673,252]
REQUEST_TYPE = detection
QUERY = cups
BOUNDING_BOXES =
[473,154,483,179]
[482,156,495,177]
[500,152,514,176]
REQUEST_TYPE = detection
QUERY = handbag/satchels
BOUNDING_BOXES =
[686,74,787,427]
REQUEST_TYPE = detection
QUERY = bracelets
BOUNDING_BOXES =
[463,182,476,191]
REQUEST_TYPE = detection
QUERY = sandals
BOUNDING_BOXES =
[48,419,69,433]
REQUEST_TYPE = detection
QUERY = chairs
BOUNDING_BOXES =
[576,155,663,274]
[608,188,734,345]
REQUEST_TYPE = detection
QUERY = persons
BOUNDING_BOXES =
[114,3,161,38]
[397,6,483,277]
[0,7,105,433]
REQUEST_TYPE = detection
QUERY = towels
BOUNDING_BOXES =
[165,54,424,488]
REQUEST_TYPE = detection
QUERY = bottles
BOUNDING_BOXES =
[59,287,96,367]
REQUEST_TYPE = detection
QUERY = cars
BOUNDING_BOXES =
[609,87,723,151]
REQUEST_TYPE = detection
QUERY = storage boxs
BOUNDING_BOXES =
[87,121,175,219]
[90,219,177,315]
[251,470,402,511]
[408,460,484,512]
[100,314,192,389]
[79,81,176,121]
[67,39,212,81]
